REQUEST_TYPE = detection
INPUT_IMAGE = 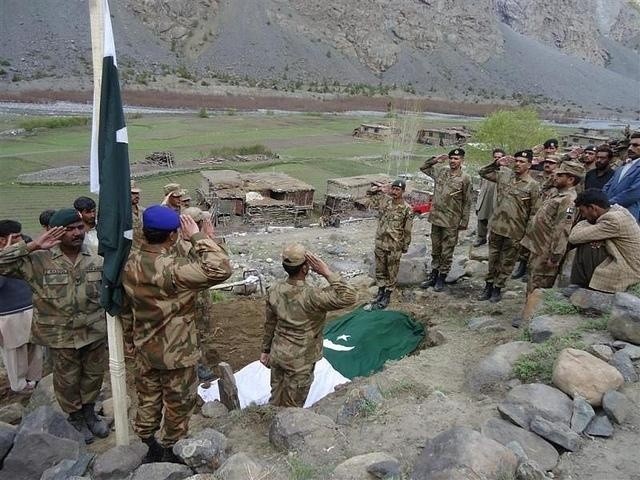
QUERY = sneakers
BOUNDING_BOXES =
[563,286,580,296]
[473,237,487,247]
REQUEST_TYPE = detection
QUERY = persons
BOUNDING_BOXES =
[39,210,56,232]
[602,132,640,227]
[477,149,543,304]
[510,155,561,282]
[260,242,357,407]
[0,220,43,395]
[511,161,584,327]
[160,183,182,214]
[0,208,109,444]
[562,189,640,298]
[584,148,616,190]
[121,205,232,464]
[557,145,598,173]
[130,180,147,228]
[74,197,97,233]
[181,189,190,208]
[184,207,214,381]
[420,148,472,291]
[473,148,505,247]
[21,235,31,242]
[366,180,413,309]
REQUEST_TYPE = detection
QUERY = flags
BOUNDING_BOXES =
[90,0,132,317]
[199,303,426,409]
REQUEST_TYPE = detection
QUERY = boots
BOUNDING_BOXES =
[523,273,529,281]
[198,363,212,379]
[512,318,520,326]
[161,448,183,462]
[491,287,501,301]
[68,411,94,443]
[511,259,527,278]
[419,269,439,288]
[84,403,109,438]
[477,282,493,301]
[434,273,447,291]
[380,290,393,304]
[142,434,162,463]
[371,287,386,304]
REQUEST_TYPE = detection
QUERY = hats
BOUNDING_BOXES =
[165,183,185,196]
[514,150,534,158]
[448,148,466,156]
[180,188,190,201]
[557,162,585,177]
[184,207,210,223]
[392,179,406,190]
[282,242,306,266]
[131,180,141,193]
[544,139,559,149]
[545,155,562,163]
[583,145,598,152]
[142,205,179,229]
[49,207,80,227]
